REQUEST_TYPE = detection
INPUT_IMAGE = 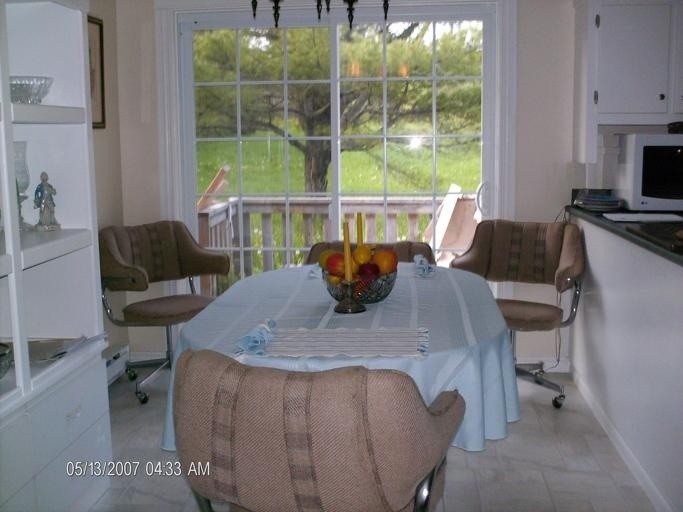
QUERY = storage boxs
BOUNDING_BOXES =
[435,192,479,253]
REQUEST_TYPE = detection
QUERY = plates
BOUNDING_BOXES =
[0,0,113,511]
[578,1,681,127]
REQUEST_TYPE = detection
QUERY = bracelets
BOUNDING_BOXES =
[323,269,396,304]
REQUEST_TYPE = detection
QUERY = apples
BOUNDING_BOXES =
[357,212,363,249]
[343,221,352,280]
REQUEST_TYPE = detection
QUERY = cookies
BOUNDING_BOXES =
[617,133,682,211]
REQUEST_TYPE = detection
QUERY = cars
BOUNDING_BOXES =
[9,75,54,103]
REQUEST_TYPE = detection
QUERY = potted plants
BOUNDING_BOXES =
[87,14,106,130]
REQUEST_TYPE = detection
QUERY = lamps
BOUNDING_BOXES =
[416,182,465,260]
[450,216,584,409]
[95,219,230,403]
[306,240,436,264]
[172,347,466,512]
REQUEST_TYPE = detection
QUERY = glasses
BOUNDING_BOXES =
[251,0,389,29]
